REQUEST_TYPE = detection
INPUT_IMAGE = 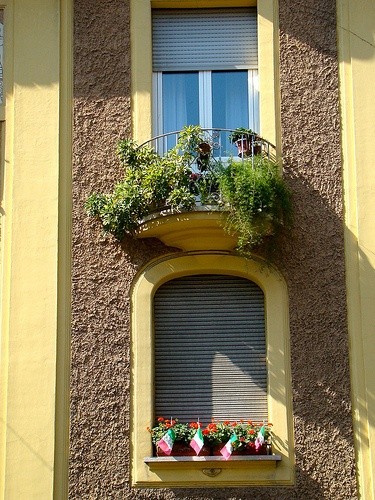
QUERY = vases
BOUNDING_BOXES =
[153,438,269,456]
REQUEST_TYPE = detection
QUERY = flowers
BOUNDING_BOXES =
[146,417,274,449]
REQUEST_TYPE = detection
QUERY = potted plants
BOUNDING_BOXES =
[85,122,295,264]
